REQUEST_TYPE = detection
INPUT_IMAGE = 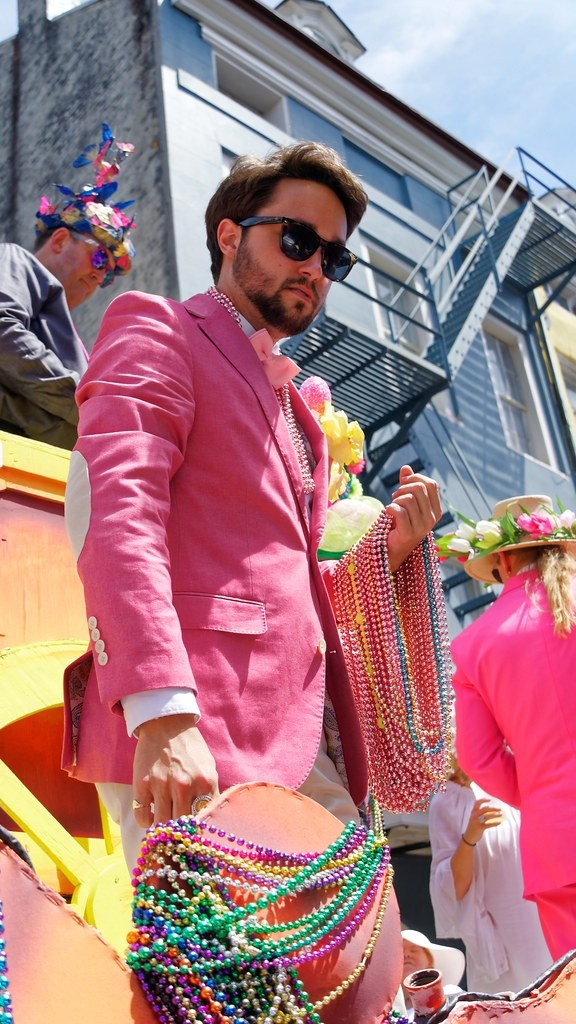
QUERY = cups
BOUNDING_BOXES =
[403,969,449,1023]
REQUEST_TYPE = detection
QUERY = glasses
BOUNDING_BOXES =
[237,214,359,283]
[68,227,115,289]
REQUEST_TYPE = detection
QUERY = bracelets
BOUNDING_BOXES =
[462,835,476,846]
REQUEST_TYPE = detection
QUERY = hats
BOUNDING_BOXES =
[435,493,576,583]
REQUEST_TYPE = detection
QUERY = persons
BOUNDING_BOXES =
[428,760,554,995]
[64,141,443,887]
[392,930,465,1024]
[448,494,576,963]
[0,198,137,452]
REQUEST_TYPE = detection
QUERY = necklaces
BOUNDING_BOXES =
[125,815,400,1024]
[206,287,316,495]
[0,900,15,1024]
[333,509,456,814]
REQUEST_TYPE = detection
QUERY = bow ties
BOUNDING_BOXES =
[249,329,302,390]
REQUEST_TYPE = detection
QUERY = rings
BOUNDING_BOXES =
[479,816,484,823]
[132,800,151,810]
[192,795,213,815]
